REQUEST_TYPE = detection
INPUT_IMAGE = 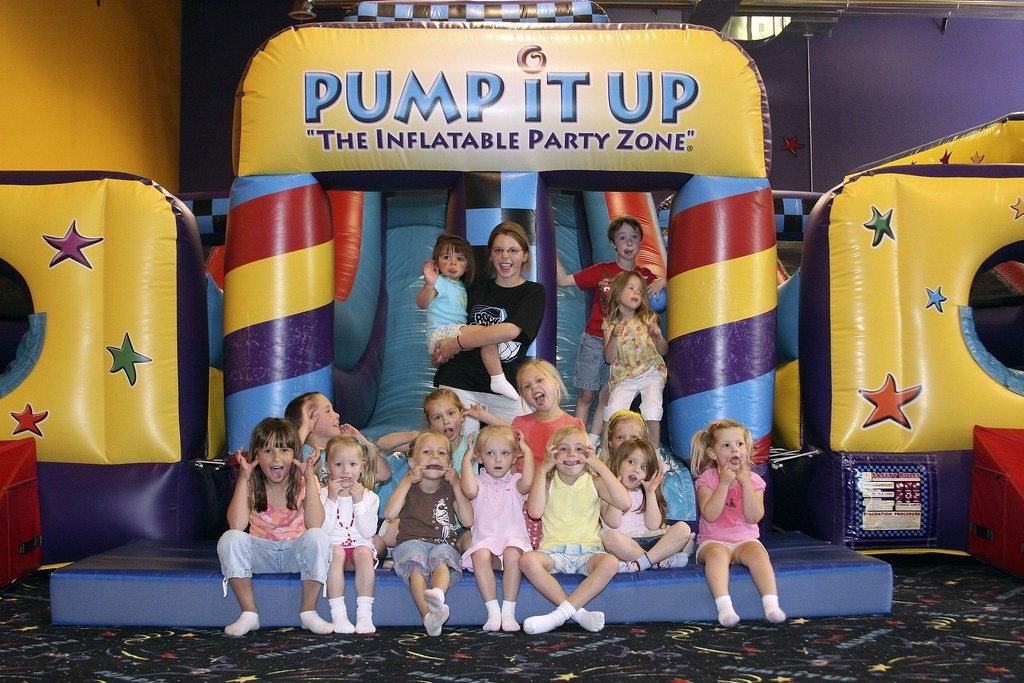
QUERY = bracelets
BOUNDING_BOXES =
[456,335,463,350]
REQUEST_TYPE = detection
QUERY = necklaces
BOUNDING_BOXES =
[336,505,356,548]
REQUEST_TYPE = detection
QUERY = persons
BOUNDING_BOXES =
[599,271,668,448]
[557,217,666,449]
[214,361,787,637]
[416,233,519,400]
[432,223,545,438]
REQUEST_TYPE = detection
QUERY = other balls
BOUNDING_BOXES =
[646,287,667,311]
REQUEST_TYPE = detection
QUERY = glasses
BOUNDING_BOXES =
[491,246,527,254]
